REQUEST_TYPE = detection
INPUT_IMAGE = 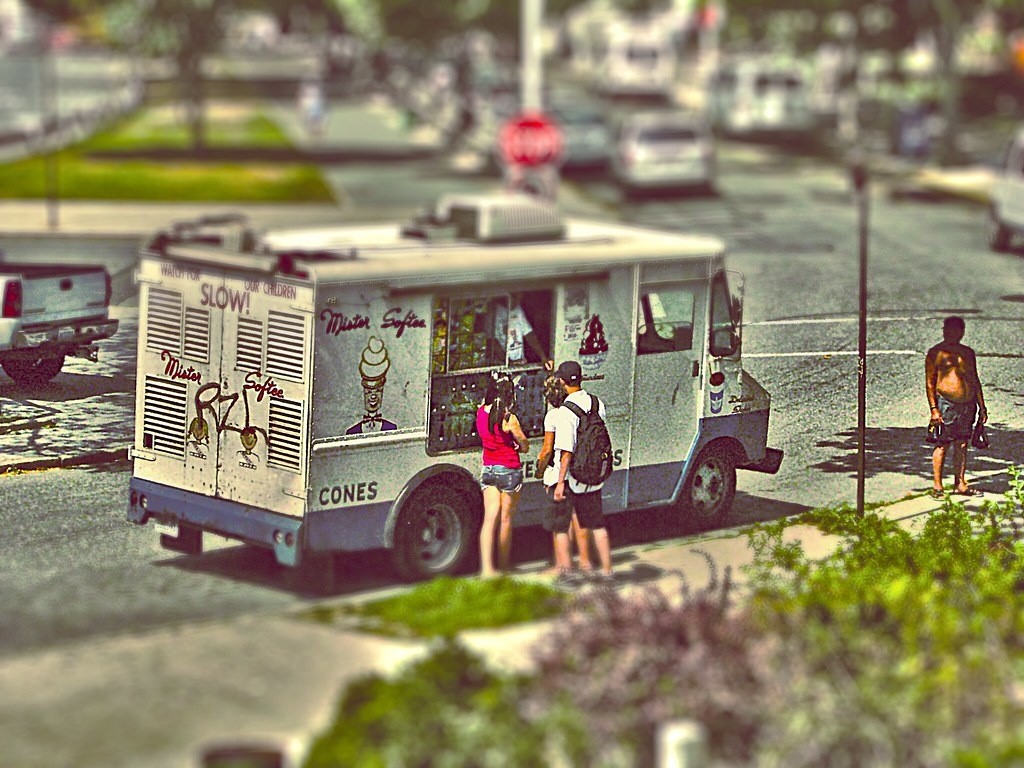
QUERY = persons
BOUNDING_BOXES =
[493,289,549,368]
[474,373,532,583]
[924,315,989,496]
[551,359,615,574]
[532,371,577,578]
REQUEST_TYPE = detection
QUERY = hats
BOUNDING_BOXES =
[554,361,581,380]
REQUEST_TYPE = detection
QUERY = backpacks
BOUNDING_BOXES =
[559,393,613,484]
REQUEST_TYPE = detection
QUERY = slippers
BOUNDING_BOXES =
[954,486,984,498]
[929,488,946,500]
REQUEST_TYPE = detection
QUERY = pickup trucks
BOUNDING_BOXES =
[1,259,120,387]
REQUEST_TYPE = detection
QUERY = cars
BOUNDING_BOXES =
[345,30,816,199]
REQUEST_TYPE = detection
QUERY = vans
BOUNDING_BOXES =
[127,189,785,597]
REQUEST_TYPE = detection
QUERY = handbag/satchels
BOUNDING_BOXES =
[971,418,990,450]
